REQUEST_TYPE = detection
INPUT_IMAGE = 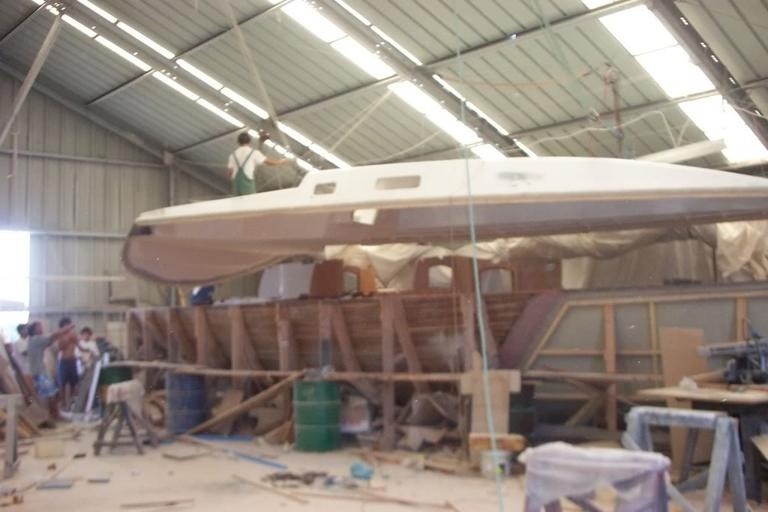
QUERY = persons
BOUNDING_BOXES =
[11,323,33,389]
[224,131,290,194]
[25,320,76,424]
[72,327,101,378]
[54,316,78,411]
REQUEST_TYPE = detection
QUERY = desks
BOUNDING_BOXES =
[635,379,768,502]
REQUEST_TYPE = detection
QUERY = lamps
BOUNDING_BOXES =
[584,0,768,170]
[35,0,538,171]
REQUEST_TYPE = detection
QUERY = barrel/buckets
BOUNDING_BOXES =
[480,451,513,479]
[292,381,342,452]
[100,367,133,417]
[166,373,205,434]
